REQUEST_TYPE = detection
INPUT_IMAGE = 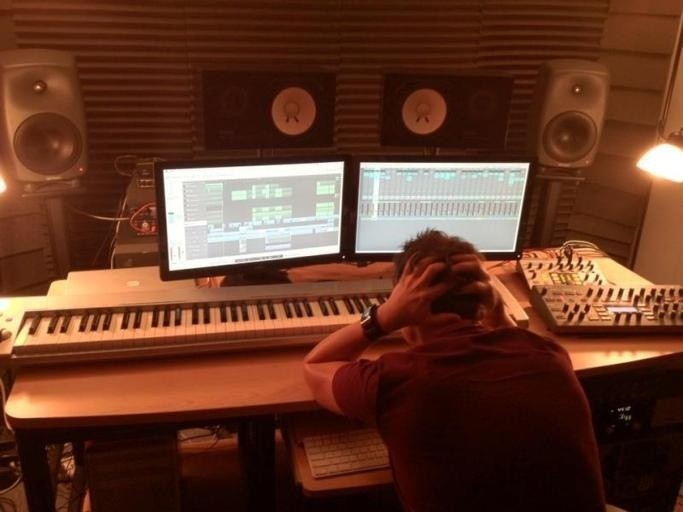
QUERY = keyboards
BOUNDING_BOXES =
[303,425,392,478]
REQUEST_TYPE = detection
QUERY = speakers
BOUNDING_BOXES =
[1,49,86,198]
[527,59,612,175]
[379,70,514,150]
[192,70,336,148]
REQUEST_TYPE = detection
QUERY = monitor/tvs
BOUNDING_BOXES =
[153,155,352,286]
[349,155,539,261]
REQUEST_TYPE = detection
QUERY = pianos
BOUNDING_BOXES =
[0,273,530,368]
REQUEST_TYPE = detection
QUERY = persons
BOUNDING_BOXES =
[303,227,606,512]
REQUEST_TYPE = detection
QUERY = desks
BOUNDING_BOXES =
[0,185,88,295]
[3,248,683,512]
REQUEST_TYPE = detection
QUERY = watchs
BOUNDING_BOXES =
[359,303,388,342]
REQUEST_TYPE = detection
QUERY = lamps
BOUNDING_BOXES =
[636,126,683,183]
